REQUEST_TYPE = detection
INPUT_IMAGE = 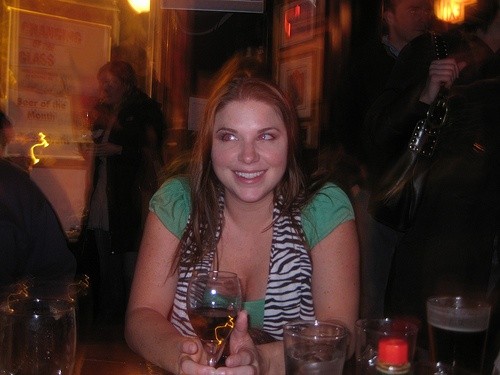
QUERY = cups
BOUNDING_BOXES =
[0,296,78,375]
[351,316,419,375]
[427,294,492,375]
[283,320,349,375]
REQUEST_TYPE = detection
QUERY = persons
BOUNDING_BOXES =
[0,108,79,299]
[78,59,166,339]
[125,56,360,375]
[321,0,500,375]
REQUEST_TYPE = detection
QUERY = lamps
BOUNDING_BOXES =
[434,0,464,24]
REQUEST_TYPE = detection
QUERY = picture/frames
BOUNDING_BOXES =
[283,0,330,37]
[277,32,331,124]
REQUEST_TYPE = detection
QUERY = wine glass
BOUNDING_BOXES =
[185,270,243,367]
